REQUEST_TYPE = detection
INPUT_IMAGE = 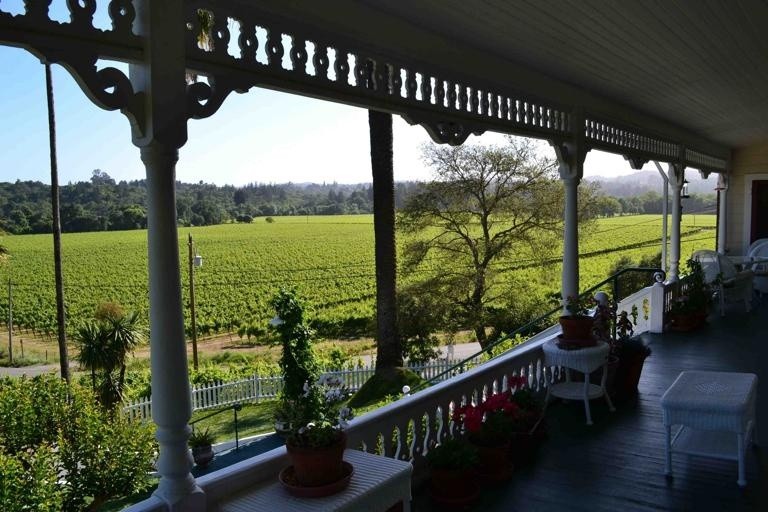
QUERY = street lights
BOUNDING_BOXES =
[186,232,202,373]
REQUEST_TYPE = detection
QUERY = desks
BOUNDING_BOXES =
[541,337,617,426]
[208,446,414,511]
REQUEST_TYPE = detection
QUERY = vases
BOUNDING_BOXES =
[512,416,547,466]
[618,359,644,395]
[607,354,620,396]
[467,436,517,485]
[423,464,482,511]
[555,315,595,347]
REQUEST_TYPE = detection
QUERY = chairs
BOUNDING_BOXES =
[690,237,768,318]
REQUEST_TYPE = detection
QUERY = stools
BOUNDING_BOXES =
[659,368,760,486]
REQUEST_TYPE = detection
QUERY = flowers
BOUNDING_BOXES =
[423,374,544,470]
[546,286,653,359]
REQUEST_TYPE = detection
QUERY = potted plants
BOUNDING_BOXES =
[268,280,358,499]
[667,258,724,333]
